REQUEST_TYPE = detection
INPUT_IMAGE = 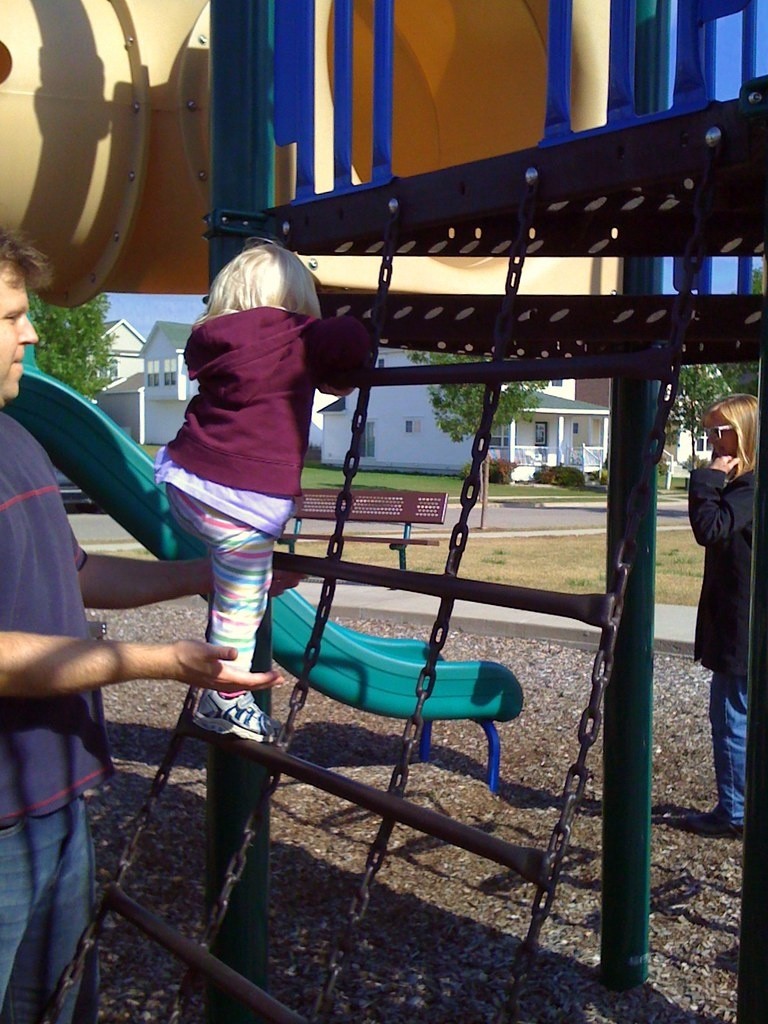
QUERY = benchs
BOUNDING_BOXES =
[279,488,449,577]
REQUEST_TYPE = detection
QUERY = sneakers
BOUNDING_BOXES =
[192,688,282,743]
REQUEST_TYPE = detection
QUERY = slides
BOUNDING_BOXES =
[5,366,525,722]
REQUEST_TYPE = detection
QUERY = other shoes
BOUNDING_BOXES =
[687,812,743,839]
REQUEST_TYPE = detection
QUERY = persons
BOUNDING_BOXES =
[1,225,307,1024]
[680,394,760,835]
[155,236,378,741]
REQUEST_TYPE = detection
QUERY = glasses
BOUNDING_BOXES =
[704,424,737,440]
